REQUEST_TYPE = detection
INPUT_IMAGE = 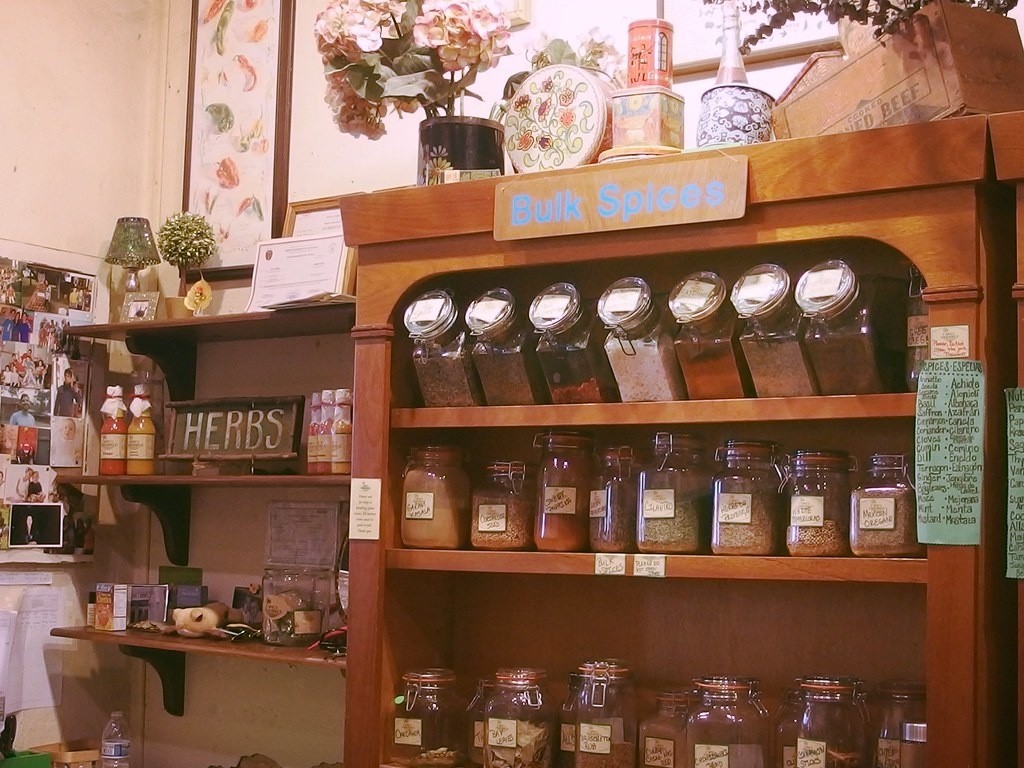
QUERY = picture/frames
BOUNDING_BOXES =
[118,291,160,324]
[657,0,842,76]
[178,0,295,282]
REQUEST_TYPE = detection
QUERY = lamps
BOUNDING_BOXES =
[104,217,161,292]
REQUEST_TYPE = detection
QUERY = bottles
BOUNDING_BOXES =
[307,392,322,474]
[404,260,938,405]
[400,424,925,556]
[332,389,351,475]
[126,384,156,475]
[100,385,126,475]
[712,4,750,85]
[318,390,336,474]
[101,710,130,768]
[261,562,332,648]
[393,656,924,768]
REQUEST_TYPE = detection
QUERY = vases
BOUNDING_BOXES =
[418,118,505,185]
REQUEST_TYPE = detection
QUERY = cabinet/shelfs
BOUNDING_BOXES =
[49,105,1024,768]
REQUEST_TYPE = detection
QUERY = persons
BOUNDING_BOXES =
[1,349,46,389]
[0,471,4,497]
[23,515,39,545]
[54,368,82,417]
[39,319,70,349]
[0,309,31,342]
[44,280,51,311]
[55,419,81,465]
[6,467,46,503]
[10,393,36,426]
[58,516,95,555]
[6,284,16,304]
[69,287,91,311]
[49,484,69,516]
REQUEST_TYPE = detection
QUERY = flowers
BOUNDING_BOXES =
[314,0,620,141]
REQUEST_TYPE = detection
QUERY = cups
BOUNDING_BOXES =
[695,87,776,149]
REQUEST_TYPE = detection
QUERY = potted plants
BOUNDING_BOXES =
[705,0,1023,138]
[156,211,216,317]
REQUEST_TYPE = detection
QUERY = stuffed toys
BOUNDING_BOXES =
[161,601,243,638]
